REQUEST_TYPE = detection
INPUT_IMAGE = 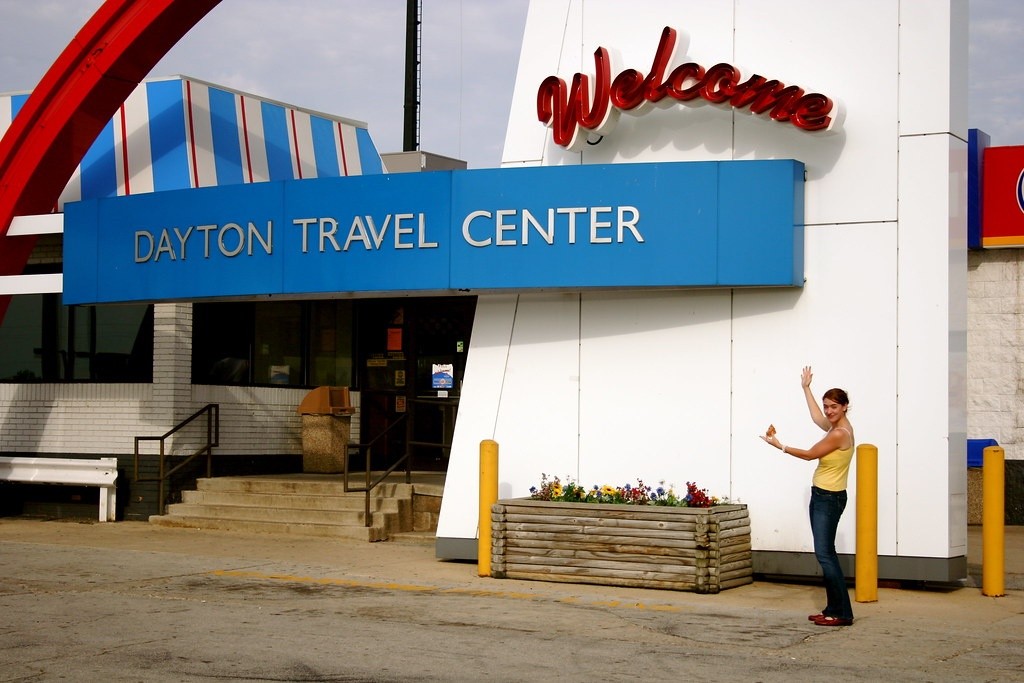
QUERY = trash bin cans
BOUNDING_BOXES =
[298,386,356,474]
[967,438,999,526]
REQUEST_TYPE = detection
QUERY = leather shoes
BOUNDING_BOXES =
[808,614,825,621]
[815,616,852,626]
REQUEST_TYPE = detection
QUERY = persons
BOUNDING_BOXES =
[759,366,854,626]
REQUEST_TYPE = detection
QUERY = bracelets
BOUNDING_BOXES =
[783,445,787,453]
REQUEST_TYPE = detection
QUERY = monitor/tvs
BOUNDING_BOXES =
[431,362,455,391]
